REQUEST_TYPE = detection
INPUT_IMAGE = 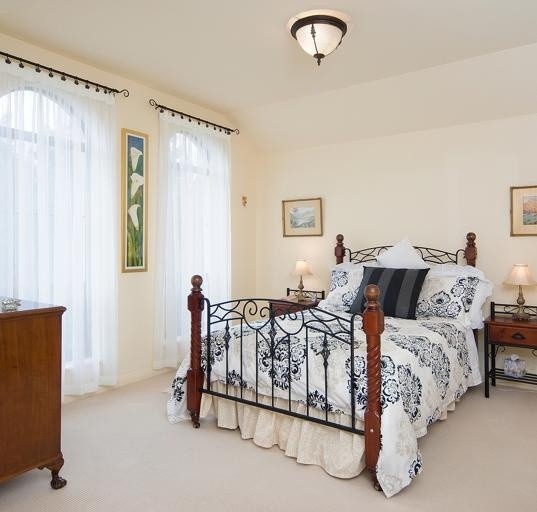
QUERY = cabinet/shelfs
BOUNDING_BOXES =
[0,293,71,493]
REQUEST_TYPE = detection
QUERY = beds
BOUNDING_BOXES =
[188,230,478,491]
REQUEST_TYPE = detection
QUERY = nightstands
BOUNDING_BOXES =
[479,301,537,401]
[267,287,326,320]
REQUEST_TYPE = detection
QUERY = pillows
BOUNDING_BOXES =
[316,239,493,330]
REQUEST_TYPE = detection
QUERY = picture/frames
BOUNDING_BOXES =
[510,185,537,236]
[118,124,151,275]
[281,198,323,237]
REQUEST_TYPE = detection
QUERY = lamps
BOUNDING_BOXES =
[289,258,318,304]
[286,9,351,67]
[500,262,535,323]
